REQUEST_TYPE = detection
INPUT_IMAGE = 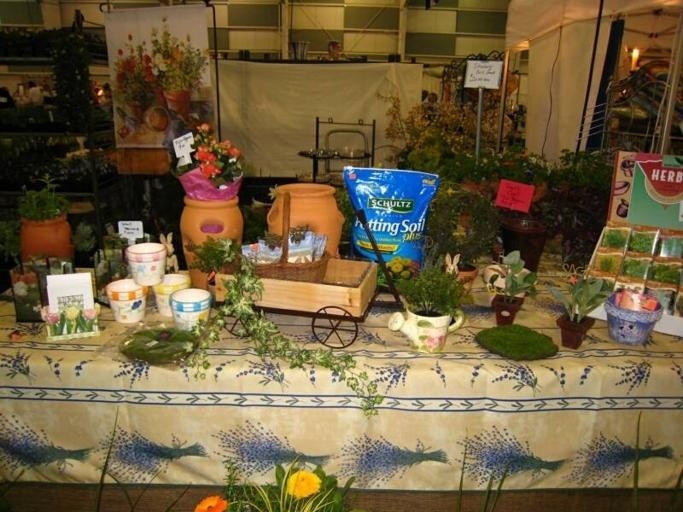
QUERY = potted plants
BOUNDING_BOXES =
[476,152,560,274]
[551,273,612,348]
[395,266,473,354]
[16,173,73,265]
[483,249,539,327]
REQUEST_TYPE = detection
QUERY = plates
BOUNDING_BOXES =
[614,182,631,195]
[120,330,201,361]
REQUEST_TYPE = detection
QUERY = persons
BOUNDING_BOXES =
[328,41,342,52]
[423,93,442,104]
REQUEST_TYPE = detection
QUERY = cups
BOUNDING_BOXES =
[606,290,664,346]
[108,242,214,332]
[292,41,312,60]
[621,159,633,177]
[144,104,171,131]
[615,199,630,218]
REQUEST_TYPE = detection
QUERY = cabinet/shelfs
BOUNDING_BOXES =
[297,116,376,184]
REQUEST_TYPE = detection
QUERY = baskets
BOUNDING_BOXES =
[242,192,330,282]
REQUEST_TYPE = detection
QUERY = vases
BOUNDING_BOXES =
[126,87,188,131]
[602,285,662,347]
[106,242,209,331]
[265,182,346,260]
[177,194,245,271]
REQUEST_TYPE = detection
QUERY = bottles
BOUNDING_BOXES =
[329,40,342,59]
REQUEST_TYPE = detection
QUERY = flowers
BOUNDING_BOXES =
[170,123,244,202]
[192,452,356,512]
[104,13,209,106]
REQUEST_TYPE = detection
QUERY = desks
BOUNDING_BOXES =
[0,291,683,490]
